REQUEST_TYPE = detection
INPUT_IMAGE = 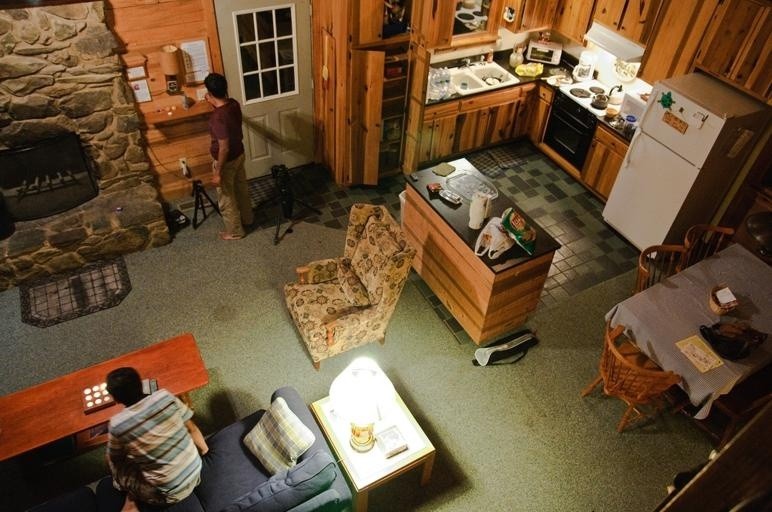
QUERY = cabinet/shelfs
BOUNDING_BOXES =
[579,120,630,204]
[498,0,560,34]
[312,0,431,191]
[635,0,720,87]
[592,0,665,49]
[452,86,522,159]
[418,100,460,171]
[427,0,507,55]
[553,0,596,48]
[696,1,772,106]
[513,82,555,145]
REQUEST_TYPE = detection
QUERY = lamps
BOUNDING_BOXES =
[159,43,182,95]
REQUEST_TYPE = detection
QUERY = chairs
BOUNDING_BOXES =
[282,203,417,372]
[635,244,689,295]
[675,224,736,274]
[669,377,771,452]
[581,316,682,434]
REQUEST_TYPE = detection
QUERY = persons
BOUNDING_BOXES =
[100,367,209,508]
[204,72,253,239]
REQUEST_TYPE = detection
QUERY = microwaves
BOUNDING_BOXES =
[525,39,563,66]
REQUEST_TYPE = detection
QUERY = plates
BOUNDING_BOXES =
[546,75,573,88]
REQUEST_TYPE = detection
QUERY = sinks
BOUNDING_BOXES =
[610,96,625,104]
[471,63,513,87]
[447,70,485,92]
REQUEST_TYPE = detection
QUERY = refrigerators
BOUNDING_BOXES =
[600,71,772,261]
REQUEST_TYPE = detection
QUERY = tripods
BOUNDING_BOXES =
[253,164,322,247]
[190,179,222,229]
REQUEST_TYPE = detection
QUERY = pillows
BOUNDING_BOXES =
[336,262,370,308]
[240,395,316,475]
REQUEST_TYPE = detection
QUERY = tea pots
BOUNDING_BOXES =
[608,85,626,106]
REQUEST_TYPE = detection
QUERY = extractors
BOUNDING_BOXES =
[582,22,645,65]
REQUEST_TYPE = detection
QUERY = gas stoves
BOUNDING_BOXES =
[559,78,624,119]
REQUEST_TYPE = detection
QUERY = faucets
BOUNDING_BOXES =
[608,83,623,99]
[460,58,473,67]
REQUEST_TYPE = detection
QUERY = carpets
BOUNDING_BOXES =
[467,147,529,180]
[18,255,132,329]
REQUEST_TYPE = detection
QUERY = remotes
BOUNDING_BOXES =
[150,379,157,394]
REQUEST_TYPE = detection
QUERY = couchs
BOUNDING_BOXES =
[27,387,352,512]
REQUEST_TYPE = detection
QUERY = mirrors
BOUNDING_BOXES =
[452,0,493,38]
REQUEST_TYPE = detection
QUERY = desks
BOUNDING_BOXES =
[310,384,436,512]
[0,333,210,462]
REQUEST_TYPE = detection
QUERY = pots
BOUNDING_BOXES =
[592,94,610,110]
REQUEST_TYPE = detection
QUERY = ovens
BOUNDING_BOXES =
[542,91,599,172]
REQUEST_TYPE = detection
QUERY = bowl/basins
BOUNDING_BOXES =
[604,107,618,119]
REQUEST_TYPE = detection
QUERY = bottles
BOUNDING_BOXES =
[432,66,452,93]
[487,49,494,64]
